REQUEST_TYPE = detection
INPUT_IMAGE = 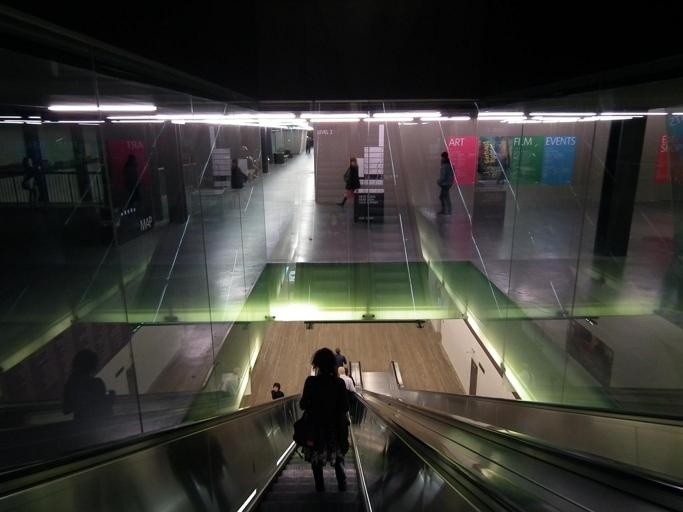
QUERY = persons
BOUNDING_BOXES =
[71,150,93,201]
[62,349,115,432]
[336,158,361,206]
[21,157,41,201]
[437,152,455,214]
[271,383,284,399]
[293,347,356,493]
[306,136,311,153]
[231,159,248,189]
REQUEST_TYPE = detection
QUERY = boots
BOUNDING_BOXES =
[337,197,346,205]
